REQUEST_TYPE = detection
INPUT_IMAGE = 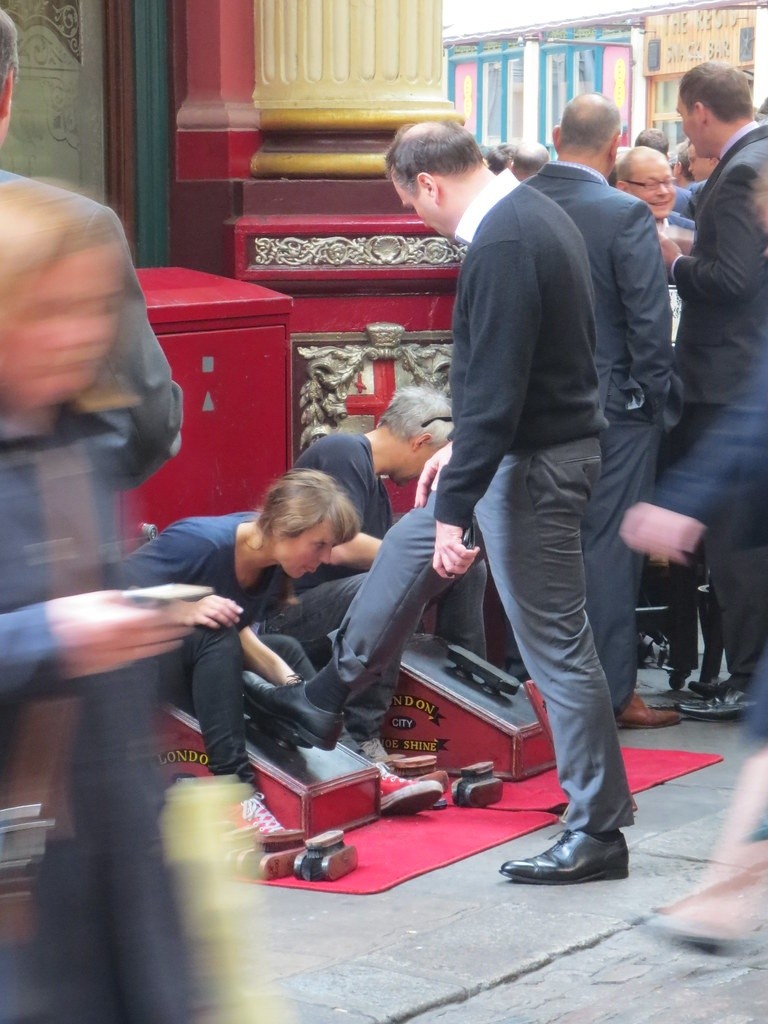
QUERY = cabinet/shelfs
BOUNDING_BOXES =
[116,267,294,553]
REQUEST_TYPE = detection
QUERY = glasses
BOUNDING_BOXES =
[625,177,677,190]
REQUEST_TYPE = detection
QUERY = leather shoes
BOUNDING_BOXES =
[615,693,681,728]
[674,681,768,723]
[241,670,344,751]
[499,830,629,885]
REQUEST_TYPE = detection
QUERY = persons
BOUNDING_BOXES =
[1,9,184,575]
[262,385,487,761]
[123,468,443,850]
[240,118,637,885]
[0,172,193,1024]
[479,62,767,943]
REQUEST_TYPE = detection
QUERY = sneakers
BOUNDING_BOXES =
[373,763,443,815]
[229,791,286,834]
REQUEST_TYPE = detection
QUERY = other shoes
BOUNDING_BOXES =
[688,677,722,699]
[338,728,389,763]
[668,670,690,689]
